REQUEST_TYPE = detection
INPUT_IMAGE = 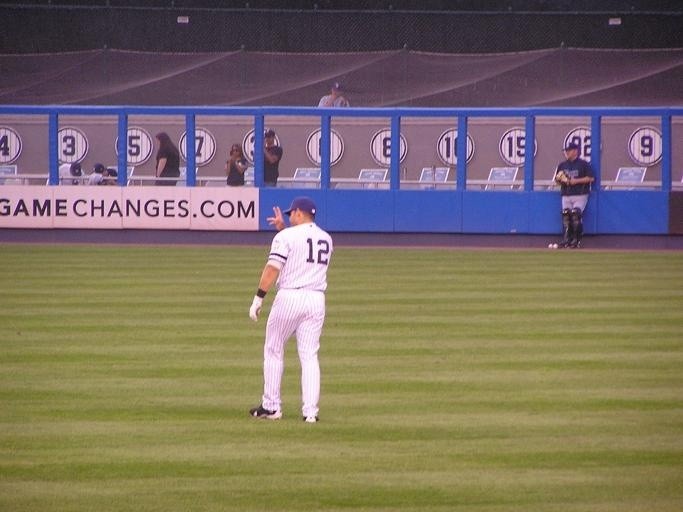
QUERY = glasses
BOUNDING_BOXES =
[232,148,239,152]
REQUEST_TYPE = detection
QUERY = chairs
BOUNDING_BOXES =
[0,165,17,185]
[177,166,198,185]
[243,167,254,185]
[292,168,321,183]
[417,166,648,191]
[359,168,388,183]
[106,166,135,185]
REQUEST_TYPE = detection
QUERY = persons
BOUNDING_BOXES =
[249,197,335,424]
[224,128,283,186]
[552,143,595,249]
[316,82,351,108]
[45,161,116,186]
[154,131,181,185]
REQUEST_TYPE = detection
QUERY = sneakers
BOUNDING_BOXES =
[559,239,581,248]
[250,405,282,419]
[303,415,319,423]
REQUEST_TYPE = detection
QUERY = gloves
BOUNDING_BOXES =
[249,294,263,321]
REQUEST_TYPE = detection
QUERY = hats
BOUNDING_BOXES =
[332,82,342,91]
[265,129,275,136]
[283,196,316,215]
[562,143,578,149]
